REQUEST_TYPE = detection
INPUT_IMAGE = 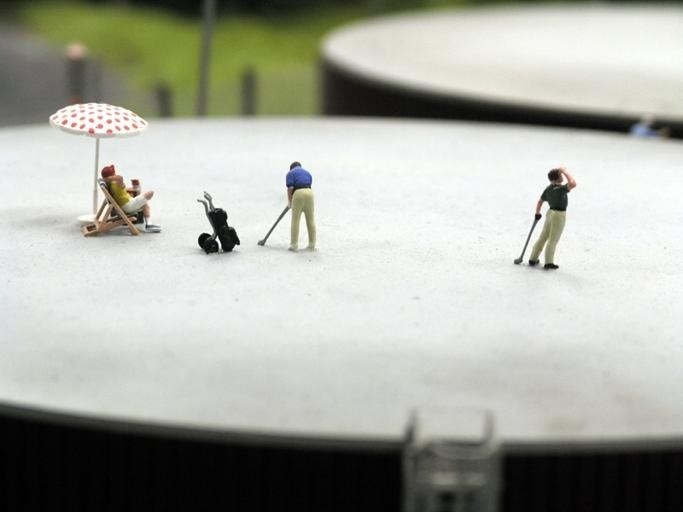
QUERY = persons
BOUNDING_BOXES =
[98,164,161,233]
[286,162,316,252]
[528,168,577,270]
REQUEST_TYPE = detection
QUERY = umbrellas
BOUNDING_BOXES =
[49,102,148,215]
